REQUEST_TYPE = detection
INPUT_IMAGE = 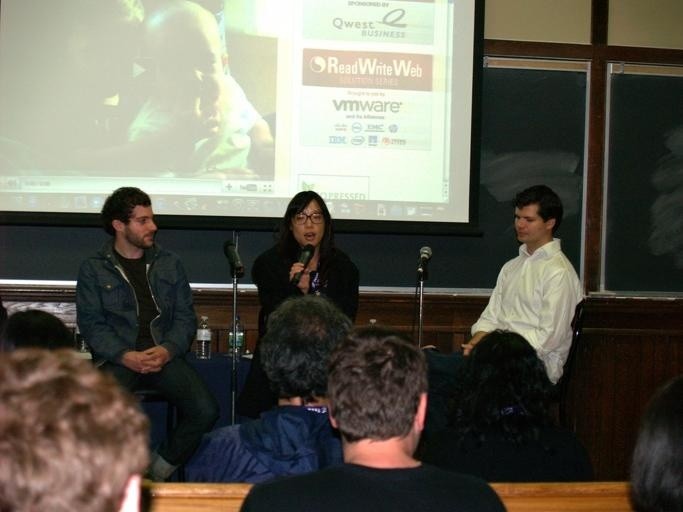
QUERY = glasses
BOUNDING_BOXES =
[295,213,324,224]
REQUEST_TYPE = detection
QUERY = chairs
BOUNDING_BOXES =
[131,383,186,483]
[553,298,587,426]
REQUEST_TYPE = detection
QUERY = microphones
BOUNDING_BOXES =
[222,240,243,270]
[416,246,432,281]
[290,243,314,286]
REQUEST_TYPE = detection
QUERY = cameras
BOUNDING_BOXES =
[306,405,327,413]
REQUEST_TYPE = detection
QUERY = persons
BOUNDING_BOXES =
[626,378,679,510]
[433,333,589,483]
[464,186,585,393]
[237,339,505,512]
[0,347,147,510]
[75,189,216,480]
[3,305,74,351]
[185,296,356,481]
[116,8,275,179]
[250,192,356,419]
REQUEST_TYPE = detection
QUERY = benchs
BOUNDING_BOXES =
[139,478,634,512]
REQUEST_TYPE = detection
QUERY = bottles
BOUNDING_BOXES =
[229,314,245,356]
[196,317,210,360]
